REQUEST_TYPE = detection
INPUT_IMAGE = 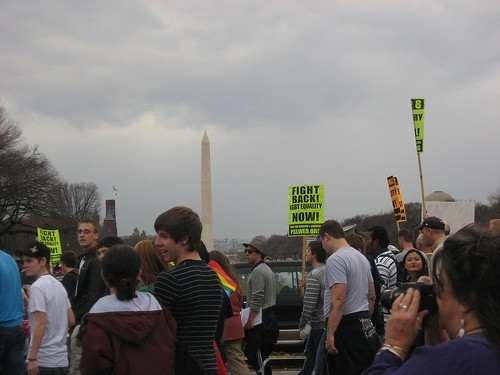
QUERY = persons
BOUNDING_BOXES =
[299,241,330,375]
[361,222,500,375]
[96,235,175,294]
[318,220,376,375]
[489,219,500,232]
[244,235,280,375]
[346,216,465,360]
[0,249,32,375]
[21,241,75,375]
[81,244,177,375]
[197,240,251,375]
[155,206,223,375]
[69,219,111,375]
[53,251,80,362]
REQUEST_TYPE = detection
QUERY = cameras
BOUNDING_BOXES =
[380,280,438,324]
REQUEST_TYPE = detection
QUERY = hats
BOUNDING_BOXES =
[15,242,50,258]
[242,235,270,255]
[415,216,445,229]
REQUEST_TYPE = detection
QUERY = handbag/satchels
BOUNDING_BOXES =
[381,255,407,284]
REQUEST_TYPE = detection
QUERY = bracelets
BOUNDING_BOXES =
[384,344,409,356]
[28,357,38,361]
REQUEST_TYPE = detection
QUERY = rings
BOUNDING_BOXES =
[398,303,409,311]
[417,318,423,324]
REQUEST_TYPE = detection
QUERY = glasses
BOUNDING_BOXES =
[244,249,257,254]
[77,230,95,234]
